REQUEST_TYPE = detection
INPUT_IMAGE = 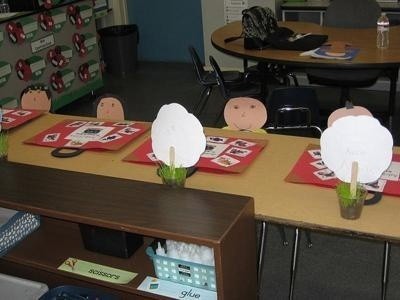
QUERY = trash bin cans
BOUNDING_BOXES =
[98,24,140,77]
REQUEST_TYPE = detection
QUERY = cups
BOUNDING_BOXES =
[156,163,188,188]
[335,183,368,220]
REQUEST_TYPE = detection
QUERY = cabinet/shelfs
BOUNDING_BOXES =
[0,159,260,300]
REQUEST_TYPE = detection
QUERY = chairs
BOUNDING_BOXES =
[188,44,324,139]
[302,1,383,108]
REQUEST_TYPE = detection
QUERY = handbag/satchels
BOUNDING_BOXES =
[224,5,275,51]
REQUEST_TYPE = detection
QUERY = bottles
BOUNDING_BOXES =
[155,239,218,292]
[376,10,391,51]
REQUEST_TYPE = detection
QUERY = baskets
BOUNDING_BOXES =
[37,283,120,300]
[0,207,42,260]
[143,239,217,294]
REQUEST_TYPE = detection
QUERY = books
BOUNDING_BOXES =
[311,46,360,60]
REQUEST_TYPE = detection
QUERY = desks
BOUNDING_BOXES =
[0,108,399,300]
[211,21,399,131]
[280,0,400,26]
[0,0,113,113]
